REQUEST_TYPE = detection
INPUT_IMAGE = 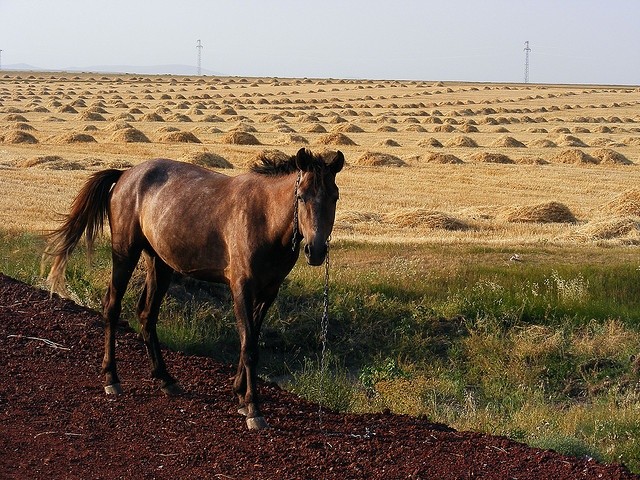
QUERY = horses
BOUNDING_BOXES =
[36,147,345,432]
